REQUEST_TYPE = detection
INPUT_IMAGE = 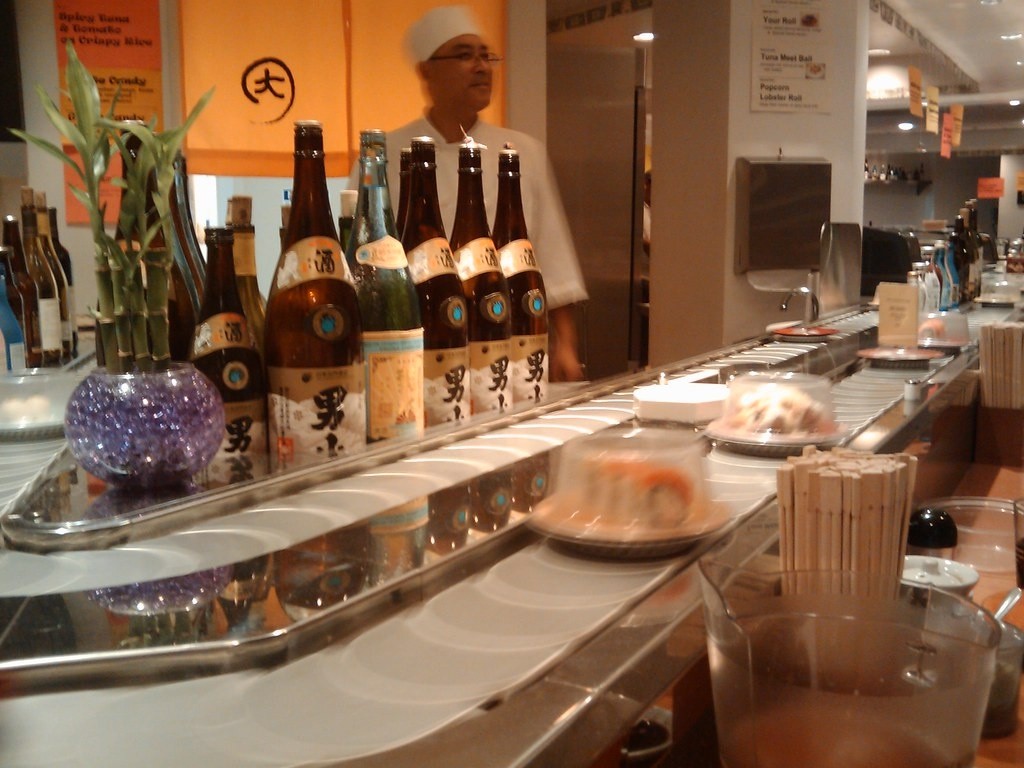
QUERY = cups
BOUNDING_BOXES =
[1013,498,1024,589]
[900,555,979,597]
[922,496,1016,608]
[982,621,1024,738]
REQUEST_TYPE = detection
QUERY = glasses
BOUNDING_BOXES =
[430,53,500,66]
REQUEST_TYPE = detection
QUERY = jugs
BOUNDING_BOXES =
[698,557,1003,768]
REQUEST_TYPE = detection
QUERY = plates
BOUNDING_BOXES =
[868,301,880,306]
[806,64,824,78]
[705,420,848,457]
[856,347,945,368]
[917,338,969,356]
[772,328,838,342]
[974,296,1021,308]
[801,14,817,27]
[528,501,733,557]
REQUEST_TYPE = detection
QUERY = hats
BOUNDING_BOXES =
[405,6,482,60]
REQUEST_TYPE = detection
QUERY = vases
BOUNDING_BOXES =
[65,362,224,484]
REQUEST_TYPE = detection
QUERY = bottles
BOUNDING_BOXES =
[887,164,924,180]
[907,198,983,320]
[907,507,957,560]
[871,164,878,180]
[879,164,886,181]
[0,186,78,376]
[94,120,547,637]
[864,159,868,181]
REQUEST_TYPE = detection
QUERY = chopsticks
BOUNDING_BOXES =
[777,445,919,600]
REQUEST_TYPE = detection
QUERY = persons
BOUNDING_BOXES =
[348,6,590,385]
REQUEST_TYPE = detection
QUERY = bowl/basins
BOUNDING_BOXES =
[623,718,672,768]
